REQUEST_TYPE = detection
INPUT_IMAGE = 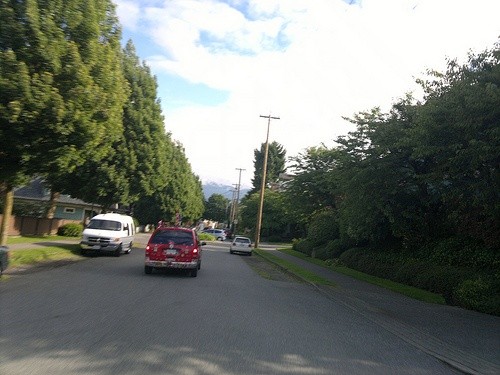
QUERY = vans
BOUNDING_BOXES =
[201,229,226,241]
[80,214,135,257]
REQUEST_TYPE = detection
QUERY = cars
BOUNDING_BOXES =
[143,226,203,277]
[224,230,232,239]
[229,237,252,256]
